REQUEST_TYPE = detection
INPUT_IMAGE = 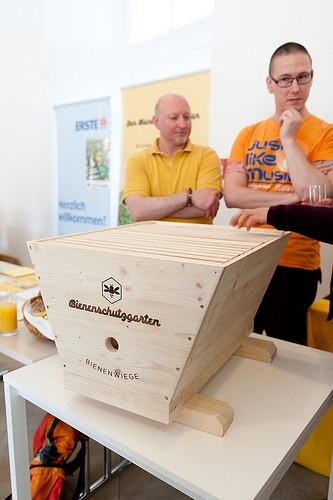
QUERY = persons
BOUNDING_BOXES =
[121,94,223,225]
[223,42,333,346]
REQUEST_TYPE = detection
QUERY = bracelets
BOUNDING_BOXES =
[187,188,192,206]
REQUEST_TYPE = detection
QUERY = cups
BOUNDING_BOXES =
[0,291,18,337]
[308,184,327,206]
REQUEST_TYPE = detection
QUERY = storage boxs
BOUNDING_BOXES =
[27,220,292,424]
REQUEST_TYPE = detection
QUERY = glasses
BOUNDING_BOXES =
[270,70,313,88]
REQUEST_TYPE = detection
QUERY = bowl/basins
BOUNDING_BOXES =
[22,295,55,342]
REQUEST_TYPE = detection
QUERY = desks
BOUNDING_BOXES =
[0,261,58,366]
[1,332,332,499]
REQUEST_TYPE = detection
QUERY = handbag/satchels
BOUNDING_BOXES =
[3,414,90,500]
